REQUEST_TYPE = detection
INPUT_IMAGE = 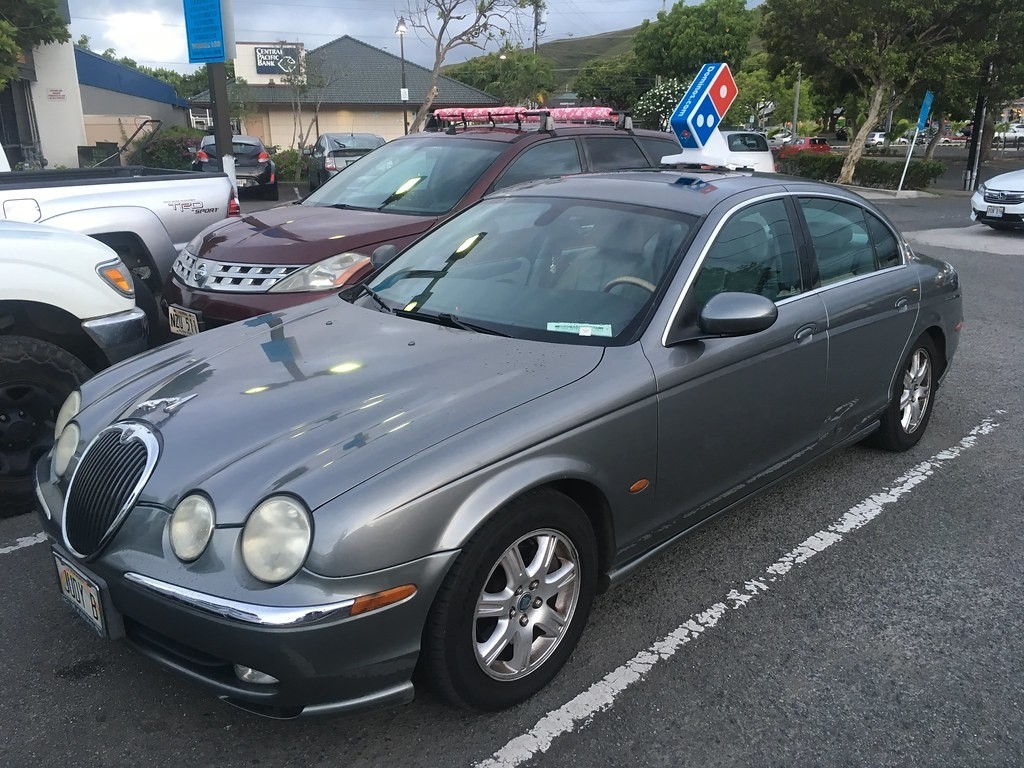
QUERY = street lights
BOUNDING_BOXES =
[394,16,409,135]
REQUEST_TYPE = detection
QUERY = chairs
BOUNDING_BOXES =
[698,221,779,300]
[552,214,653,303]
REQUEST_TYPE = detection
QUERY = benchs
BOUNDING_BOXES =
[660,222,875,292]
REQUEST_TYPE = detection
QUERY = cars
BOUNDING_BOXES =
[0,217,150,522]
[864,131,909,148]
[774,136,832,159]
[302,133,388,195]
[836,126,852,141]
[756,125,799,146]
[186,134,277,201]
[34,165,966,724]
[970,169,1024,231]
[898,121,975,146]
[991,122,1024,146]
[720,131,777,176]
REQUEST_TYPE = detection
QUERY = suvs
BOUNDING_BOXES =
[159,110,719,335]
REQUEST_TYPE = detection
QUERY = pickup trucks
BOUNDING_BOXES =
[0,141,240,352]
